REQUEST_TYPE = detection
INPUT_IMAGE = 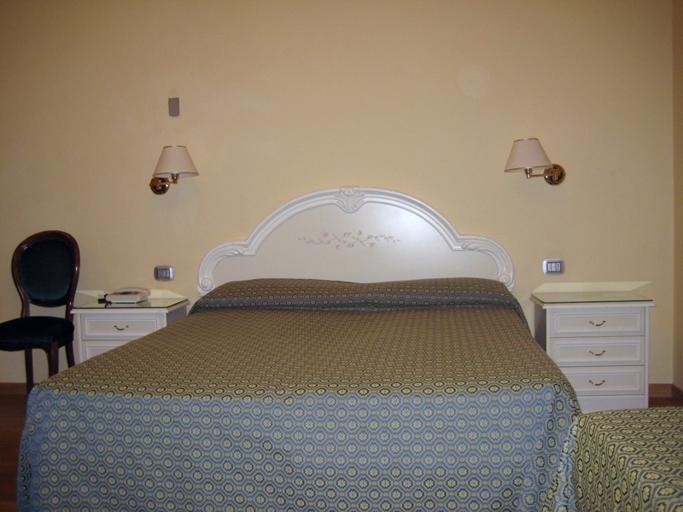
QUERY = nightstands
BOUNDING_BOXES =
[529,289,657,411]
[71,296,189,364]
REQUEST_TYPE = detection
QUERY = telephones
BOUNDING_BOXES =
[106,287,150,303]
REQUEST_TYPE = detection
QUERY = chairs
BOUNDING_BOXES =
[0,227,80,391]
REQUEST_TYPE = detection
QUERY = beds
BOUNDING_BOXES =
[15,185,582,511]
[567,401,680,512]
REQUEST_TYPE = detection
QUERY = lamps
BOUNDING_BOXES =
[148,144,201,197]
[502,136,568,186]
